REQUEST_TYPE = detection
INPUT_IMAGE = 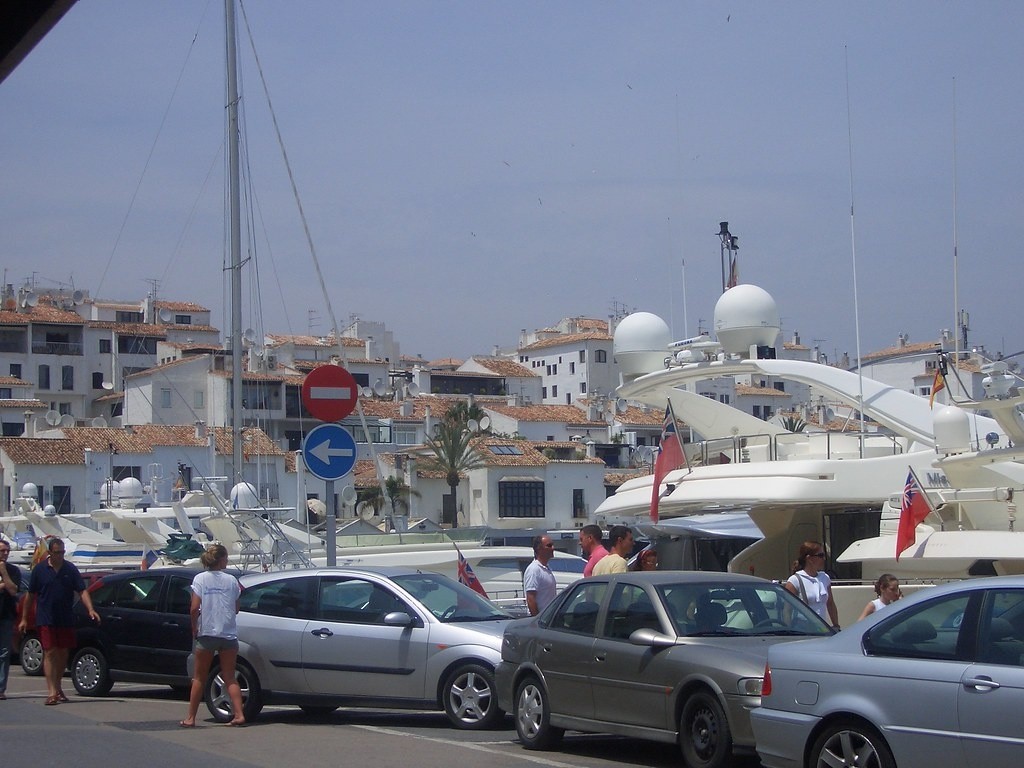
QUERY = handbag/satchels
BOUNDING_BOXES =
[790,573,816,633]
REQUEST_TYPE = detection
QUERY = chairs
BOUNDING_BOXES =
[257,593,297,618]
[568,602,600,634]
[171,588,191,603]
[696,602,727,632]
[615,602,665,640]
[989,618,1024,665]
[120,585,140,608]
[143,583,161,610]
[368,592,389,623]
[891,620,948,653]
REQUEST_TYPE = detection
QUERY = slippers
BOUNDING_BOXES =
[178,721,194,729]
[224,720,246,727]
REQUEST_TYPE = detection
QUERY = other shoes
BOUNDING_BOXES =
[0,692,6,700]
[55,691,70,703]
[45,694,58,705]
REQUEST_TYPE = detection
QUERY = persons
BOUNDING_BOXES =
[578,525,609,602]
[592,526,635,608]
[0,539,21,700]
[179,545,245,728]
[784,540,840,632]
[632,550,657,603]
[524,534,556,618]
[17,539,101,704]
[858,574,903,621]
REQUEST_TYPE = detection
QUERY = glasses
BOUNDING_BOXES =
[811,553,825,558]
[545,543,553,547]
[51,550,65,555]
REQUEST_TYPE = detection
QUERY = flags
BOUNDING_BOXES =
[930,368,945,411]
[896,470,931,562]
[32,540,47,562]
[649,403,684,523]
[457,552,489,610]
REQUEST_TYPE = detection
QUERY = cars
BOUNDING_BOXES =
[10,570,139,676]
[185,563,520,735]
[493,570,842,767]
[749,573,1024,768]
[8,563,32,665]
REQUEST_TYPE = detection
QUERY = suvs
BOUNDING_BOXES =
[65,566,262,703]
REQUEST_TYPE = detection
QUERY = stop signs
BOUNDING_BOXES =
[300,364,358,422]
